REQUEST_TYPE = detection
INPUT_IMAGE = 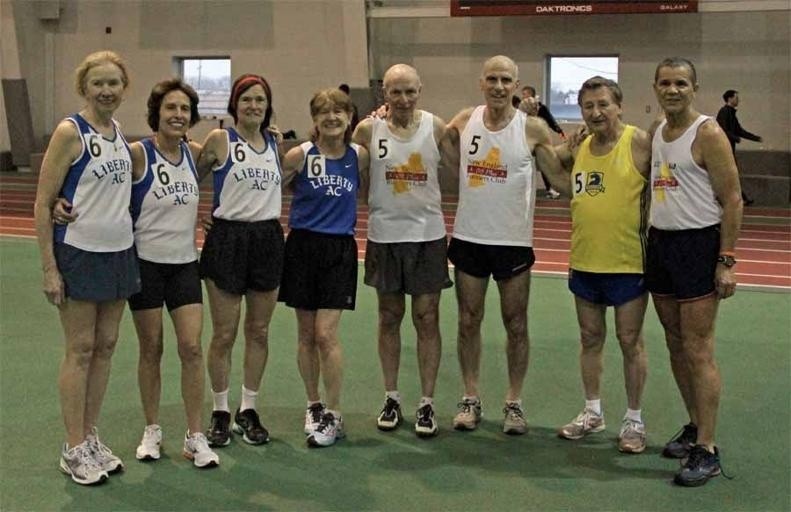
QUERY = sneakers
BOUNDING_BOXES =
[547,189,561,198]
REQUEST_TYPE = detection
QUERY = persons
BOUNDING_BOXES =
[53,78,285,480]
[520,85,568,199]
[518,76,655,456]
[199,74,297,444]
[570,57,743,485]
[32,51,141,486]
[338,84,359,142]
[368,54,570,434]
[199,87,374,446]
[353,62,463,436]
[717,89,762,206]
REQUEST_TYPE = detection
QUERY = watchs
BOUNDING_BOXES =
[718,254,736,267]
